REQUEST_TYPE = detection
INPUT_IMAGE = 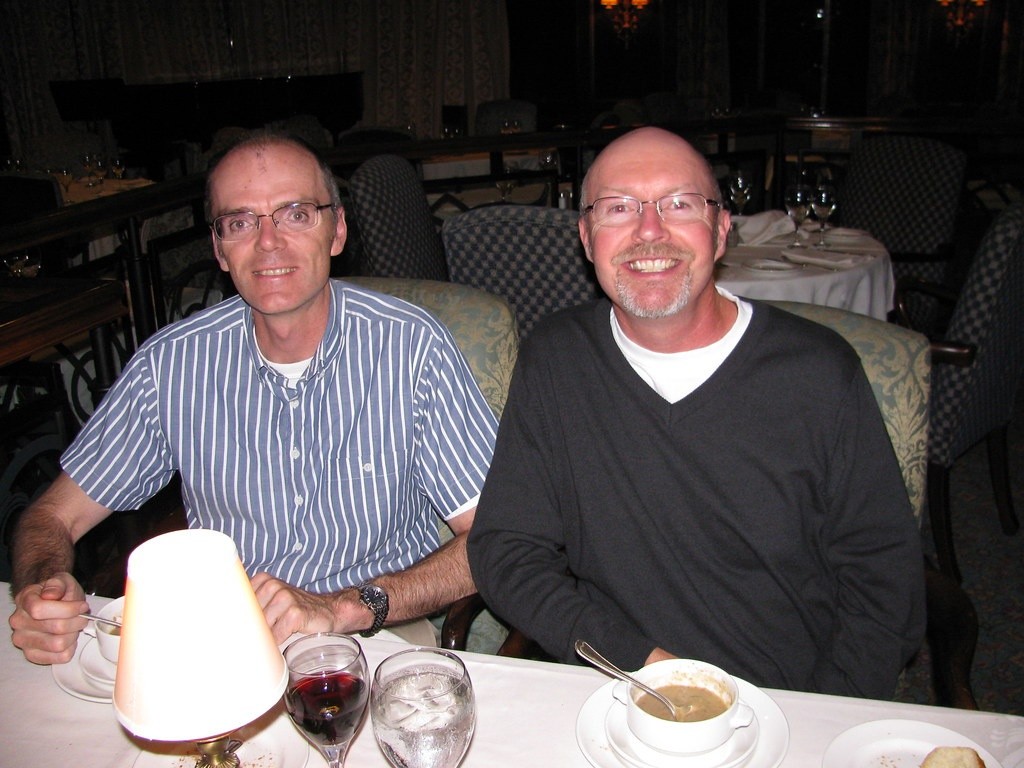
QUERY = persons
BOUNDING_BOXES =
[8,140,500,667]
[464,127,927,702]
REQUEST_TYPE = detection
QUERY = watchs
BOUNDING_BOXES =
[341,583,390,640]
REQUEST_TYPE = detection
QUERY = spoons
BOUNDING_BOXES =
[574,638,705,722]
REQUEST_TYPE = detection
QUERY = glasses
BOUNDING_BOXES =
[585,192,720,227]
[213,202,335,241]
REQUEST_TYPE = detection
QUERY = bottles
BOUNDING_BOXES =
[559,192,567,210]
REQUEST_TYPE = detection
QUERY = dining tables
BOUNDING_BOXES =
[2,579,1024,767]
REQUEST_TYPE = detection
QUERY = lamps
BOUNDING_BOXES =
[600,1,647,45]
[940,0,985,45]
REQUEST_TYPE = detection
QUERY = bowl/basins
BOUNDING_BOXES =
[612,658,754,754]
[93,594,125,664]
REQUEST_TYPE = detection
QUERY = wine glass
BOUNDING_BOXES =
[785,184,812,249]
[1,152,127,286]
[811,185,837,247]
[727,171,752,216]
[496,159,516,204]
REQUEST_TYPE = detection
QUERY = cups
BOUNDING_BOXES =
[369,647,477,768]
[512,119,521,134]
[282,632,371,768]
[439,126,459,141]
[407,120,416,136]
[500,119,513,135]
[711,104,741,118]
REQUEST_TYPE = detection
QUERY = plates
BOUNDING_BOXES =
[575,671,790,768]
[51,626,113,704]
[823,718,1003,768]
[604,691,760,768]
[78,636,116,684]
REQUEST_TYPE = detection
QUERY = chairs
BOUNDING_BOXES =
[1,100,1024,718]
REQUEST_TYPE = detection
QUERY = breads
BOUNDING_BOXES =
[918,745,986,768]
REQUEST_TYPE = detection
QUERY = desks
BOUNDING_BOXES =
[717,214,895,321]
